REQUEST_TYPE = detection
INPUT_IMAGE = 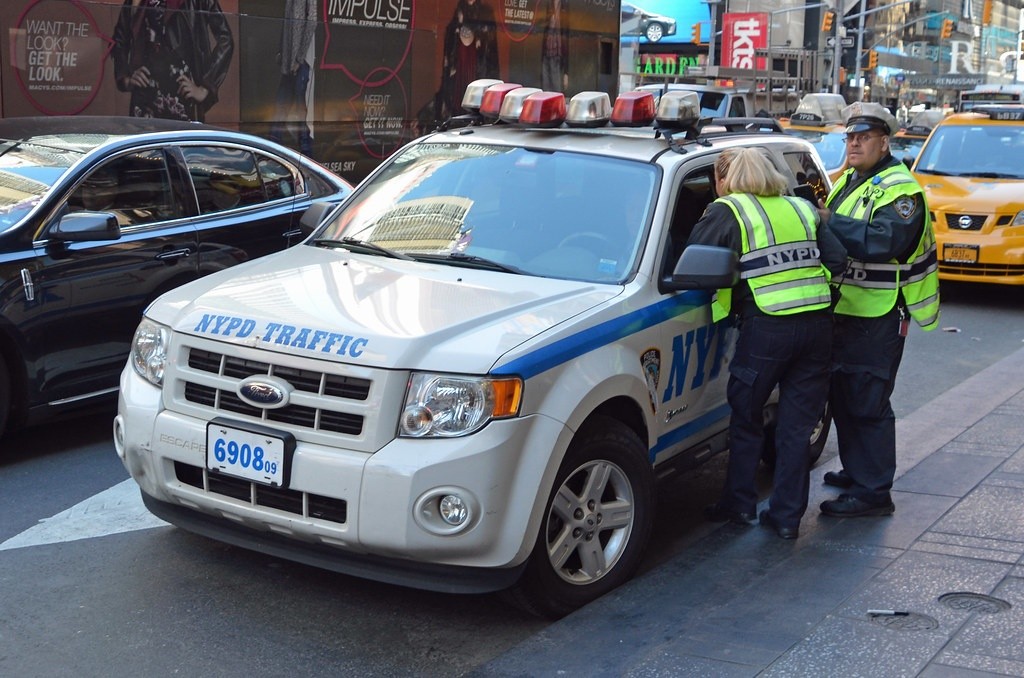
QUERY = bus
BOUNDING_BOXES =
[958,85,1024,115]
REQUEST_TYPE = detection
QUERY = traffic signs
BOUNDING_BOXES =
[826,36,854,48]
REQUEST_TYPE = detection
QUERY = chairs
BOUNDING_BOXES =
[959,128,1001,171]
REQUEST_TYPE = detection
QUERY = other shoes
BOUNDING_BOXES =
[758,508,798,539]
[820,492,895,518]
[704,503,754,528]
[824,469,855,489]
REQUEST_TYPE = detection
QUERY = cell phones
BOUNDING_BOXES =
[793,185,821,210]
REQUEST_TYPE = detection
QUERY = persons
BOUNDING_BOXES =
[80,168,137,229]
[441,0,499,128]
[268,0,318,156]
[540,0,569,92]
[816,101,940,518]
[690,147,849,540]
[112,0,234,124]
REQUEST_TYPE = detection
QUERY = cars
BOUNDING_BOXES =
[888,126,935,169]
[911,104,1024,287]
[0,113,355,455]
[621,3,677,42]
[772,116,856,185]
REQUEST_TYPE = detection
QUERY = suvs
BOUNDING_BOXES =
[112,75,839,617]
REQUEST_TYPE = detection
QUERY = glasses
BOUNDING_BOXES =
[842,134,884,145]
[81,182,117,190]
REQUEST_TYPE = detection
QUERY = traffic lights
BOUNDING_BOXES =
[983,0,994,24]
[869,49,879,68]
[691,22,701,44]
[941,19,953,39]
[822,11,834,31]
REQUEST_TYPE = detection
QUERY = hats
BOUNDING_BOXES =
[841,101,901,137]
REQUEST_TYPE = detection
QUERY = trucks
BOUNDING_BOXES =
[632,82,776,135]
